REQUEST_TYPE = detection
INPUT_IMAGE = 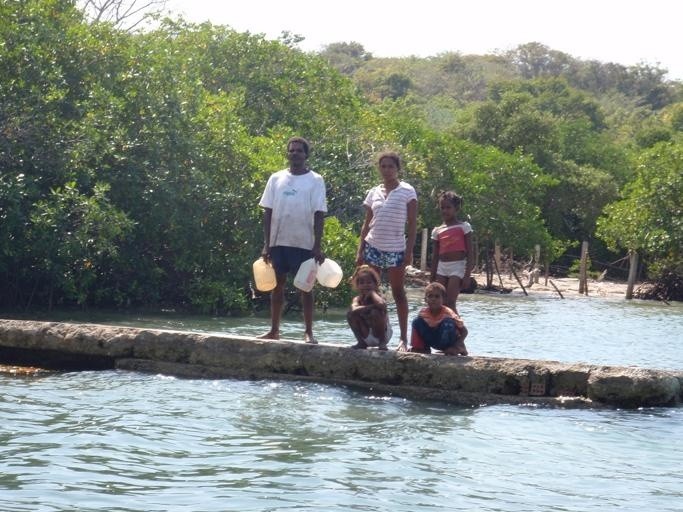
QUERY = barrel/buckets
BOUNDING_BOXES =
[316,259,343,290]
[252,257,277,292]
[294,259,318,293]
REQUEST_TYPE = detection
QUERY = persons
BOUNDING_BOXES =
[354,152,418,352]
[346,264,393,349]
[430,191,473,356]
[407,282,468,357]
[257,136,328,343]
